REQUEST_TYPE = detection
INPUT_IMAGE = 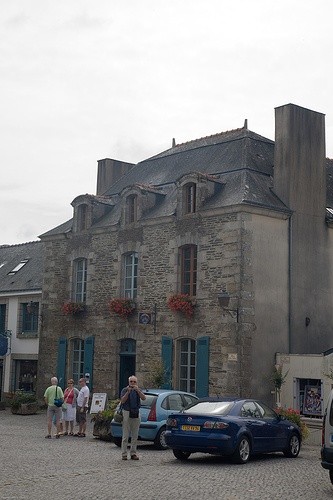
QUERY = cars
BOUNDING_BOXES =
[163,395,303,465]
[110,389,200,451]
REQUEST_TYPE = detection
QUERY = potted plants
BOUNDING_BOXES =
[9,388,39,414]
[91,399,121,438]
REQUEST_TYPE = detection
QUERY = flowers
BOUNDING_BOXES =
[108,297,136,321]
[65,302,85,314]
[167,292,196,318]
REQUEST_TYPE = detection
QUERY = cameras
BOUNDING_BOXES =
[129,385,133,388]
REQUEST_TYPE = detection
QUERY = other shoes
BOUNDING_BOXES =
[69,432,74,436]
[64,431,68,436]
[122,456,127,460]
[131,454,139,460]
[45,435,52,439]
[55,433,60,439]
[74,432,86,437]
[59,432,64,436]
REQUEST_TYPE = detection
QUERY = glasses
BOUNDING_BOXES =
[68,383,73,384]
[130,381,137,383]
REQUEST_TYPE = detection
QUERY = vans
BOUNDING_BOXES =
[321,388,333,484]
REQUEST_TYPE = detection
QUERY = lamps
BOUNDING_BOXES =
[26,300,42,323]
[217,290,239,322]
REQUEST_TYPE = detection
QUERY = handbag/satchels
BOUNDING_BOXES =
[113,410,123,423]
[62,403,67,411]
[54,398,63,407]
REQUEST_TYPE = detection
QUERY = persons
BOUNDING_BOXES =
[120,375,146,460]
[74,378,90,437]
[62,379,80,435]
[44,377,64,439]
